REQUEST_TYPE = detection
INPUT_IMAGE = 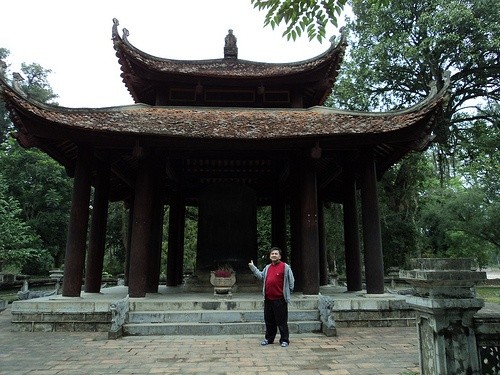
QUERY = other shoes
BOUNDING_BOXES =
[281,342,289,347]
[261,340,272,345]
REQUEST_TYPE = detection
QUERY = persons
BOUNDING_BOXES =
[248,247,295,346]
